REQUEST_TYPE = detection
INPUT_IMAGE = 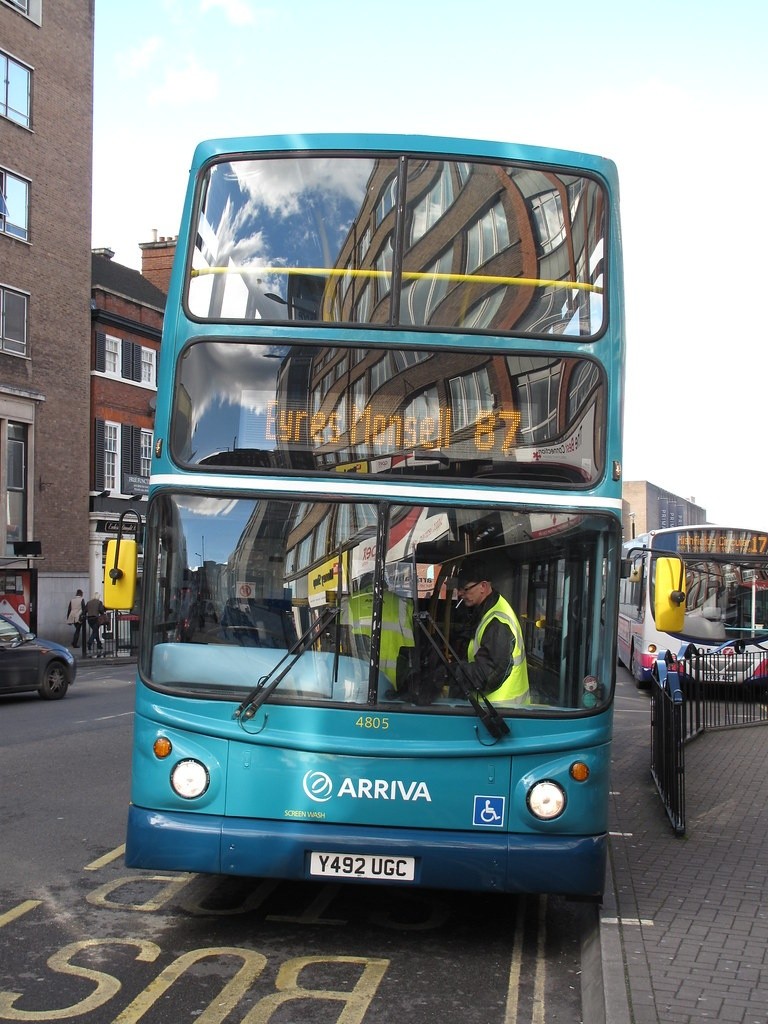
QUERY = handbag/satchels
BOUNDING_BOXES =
[98,613,109,624]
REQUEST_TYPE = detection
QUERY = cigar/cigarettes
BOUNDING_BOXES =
[456,599,463,608]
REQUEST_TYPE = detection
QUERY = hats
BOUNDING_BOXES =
[444,557,495,589]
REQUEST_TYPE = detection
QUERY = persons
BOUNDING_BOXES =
[336,573,415,691]
[67,589,85,648]
[83,592,105,652]
[441,558,531,706]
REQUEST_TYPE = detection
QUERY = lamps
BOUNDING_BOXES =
[99,490,111,497]
[131,494,142,501]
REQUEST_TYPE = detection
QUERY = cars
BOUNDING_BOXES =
[1,614,77,700]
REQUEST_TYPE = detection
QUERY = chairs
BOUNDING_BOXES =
[224,597,297,648]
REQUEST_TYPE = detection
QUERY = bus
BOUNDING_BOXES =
[102,132,686,914]
[562,526,768,689]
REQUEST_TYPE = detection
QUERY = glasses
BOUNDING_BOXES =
[459,582,481,591]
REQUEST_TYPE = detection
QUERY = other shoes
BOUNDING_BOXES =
[87,642,91,650]
[98,646,103,649]
[74,645,80,648]
